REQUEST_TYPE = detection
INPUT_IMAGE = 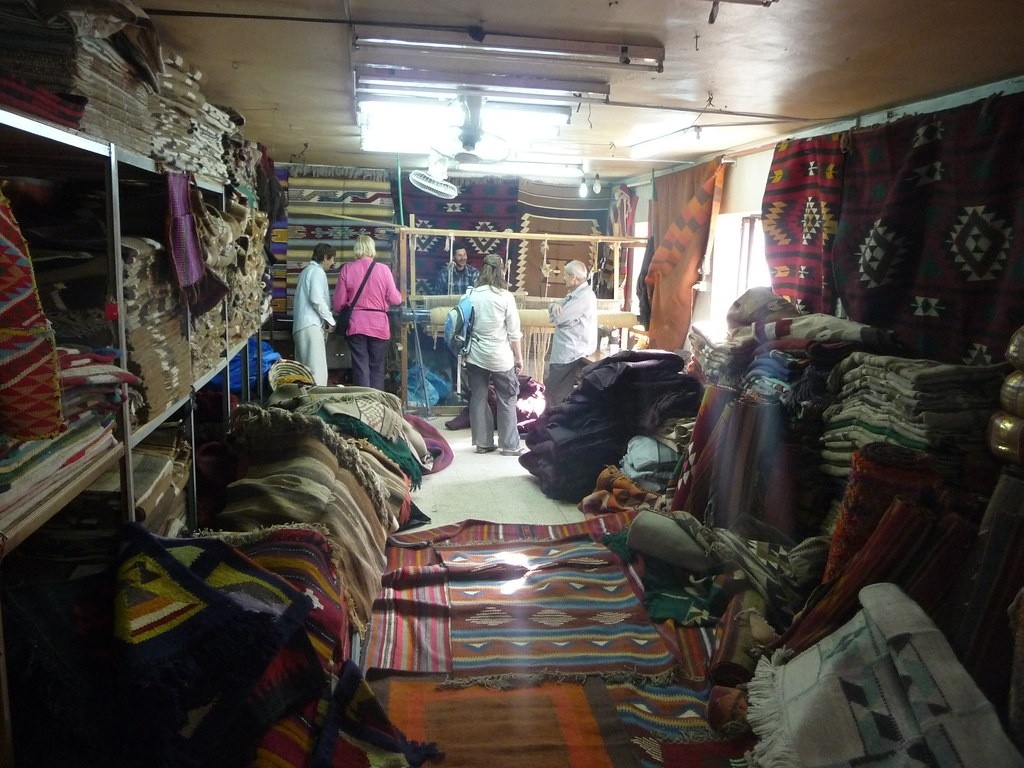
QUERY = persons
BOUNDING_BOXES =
[443,253,531,455]
[332,234,403,391]
[293,243,336,386]
[433,243,481,294]
[539,260,598,420]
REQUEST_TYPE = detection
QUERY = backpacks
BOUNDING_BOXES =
[443,285,475,359]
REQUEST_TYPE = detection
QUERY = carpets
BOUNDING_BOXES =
[606,626,756,768]
[359,507,685,686]
[433,532,682,692]
[366,668,642,768]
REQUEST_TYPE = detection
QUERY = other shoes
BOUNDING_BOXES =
[501,446,533,456]
[476,444,498,454]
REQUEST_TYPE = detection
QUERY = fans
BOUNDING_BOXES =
[430,97,511,165]
[409,153,459,199]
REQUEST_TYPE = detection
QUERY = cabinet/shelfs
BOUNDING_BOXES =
[0,109,274,768]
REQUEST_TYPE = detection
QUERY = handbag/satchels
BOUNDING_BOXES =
[335,305,352,338]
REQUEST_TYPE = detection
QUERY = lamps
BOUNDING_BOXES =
[353,94,572,124]
[351,24,665,74]
[352,67,611,107]
